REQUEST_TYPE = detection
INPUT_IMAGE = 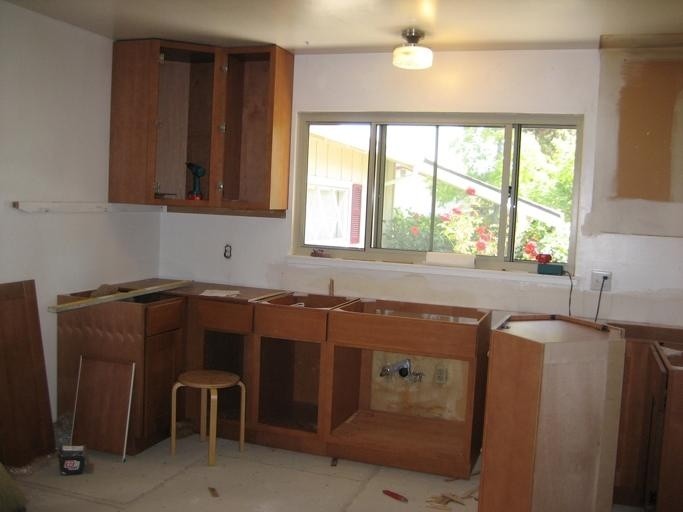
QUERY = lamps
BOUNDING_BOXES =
[392,27,433,70]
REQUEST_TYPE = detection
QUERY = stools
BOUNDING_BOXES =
[170,372,248,463]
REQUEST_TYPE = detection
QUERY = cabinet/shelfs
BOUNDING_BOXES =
[247,292,358,457]
[615,321,683,511]
[480,308,624,511]
[326,298,491,479]
[106,38,295,216]
[189,285,284,446]
[54,275,186,457]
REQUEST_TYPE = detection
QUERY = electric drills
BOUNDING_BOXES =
[185,162,206,200]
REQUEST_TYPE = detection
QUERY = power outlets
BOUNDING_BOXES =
[590,271,613,294]
